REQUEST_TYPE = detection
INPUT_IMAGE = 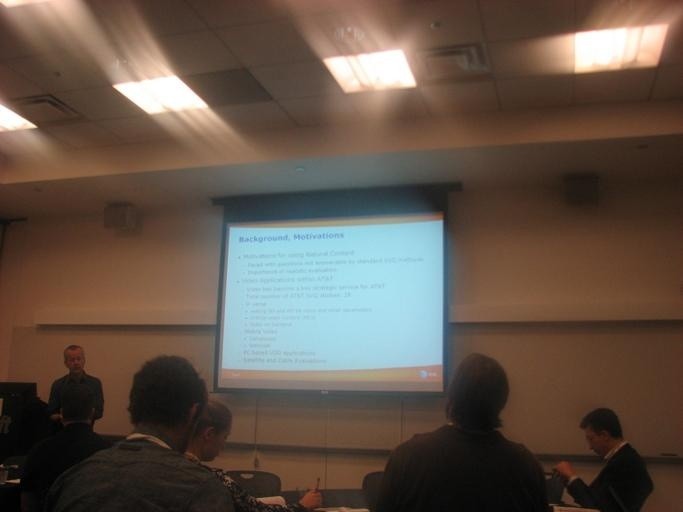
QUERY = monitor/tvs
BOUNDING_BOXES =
[0,382,37,398]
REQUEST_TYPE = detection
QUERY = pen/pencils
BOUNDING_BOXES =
[315,475,321,494]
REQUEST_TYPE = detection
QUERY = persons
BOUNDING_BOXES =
[48,347,105,433]
[44,355,236,512]
[22,384,112,512]
[552,408,653,512]
[187,400,322,512]
[375,353,548,512]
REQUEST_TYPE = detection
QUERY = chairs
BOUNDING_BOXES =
[224,468,281,497]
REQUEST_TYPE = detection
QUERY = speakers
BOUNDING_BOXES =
[103,206,138,229]
[561,173,600,206]
